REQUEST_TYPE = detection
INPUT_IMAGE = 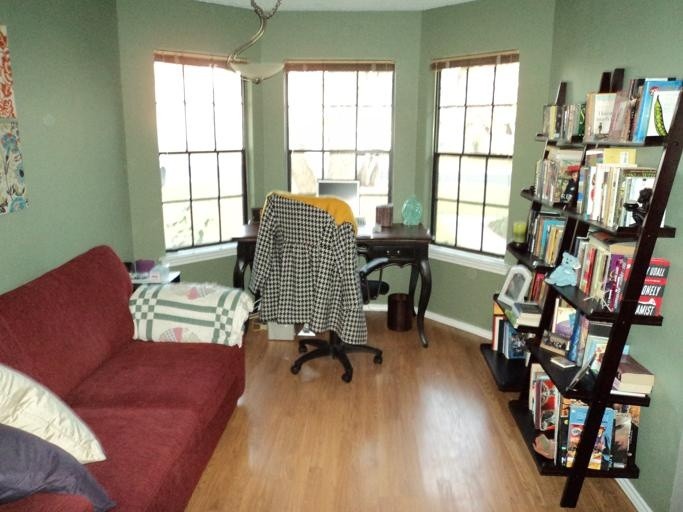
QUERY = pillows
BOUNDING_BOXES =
[0,361,107,466]
[0,422,118,512]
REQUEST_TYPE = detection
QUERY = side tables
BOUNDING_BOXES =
[128,271,182,294]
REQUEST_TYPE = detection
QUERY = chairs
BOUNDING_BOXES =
[269,192,384,384]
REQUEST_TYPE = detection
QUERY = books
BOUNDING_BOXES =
[533,77,681,231]
[492,313,641,471]
[504,209,671,397]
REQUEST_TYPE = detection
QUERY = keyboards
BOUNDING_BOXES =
[356,226,372,239]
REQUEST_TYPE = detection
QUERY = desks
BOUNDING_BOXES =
[230,223,434,349]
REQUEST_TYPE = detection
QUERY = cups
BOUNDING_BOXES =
[513,221,527,244]
[376,206,392,228]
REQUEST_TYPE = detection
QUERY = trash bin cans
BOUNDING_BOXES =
[387,293,412,332]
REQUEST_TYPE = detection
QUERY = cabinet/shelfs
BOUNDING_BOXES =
[478,68,683,508]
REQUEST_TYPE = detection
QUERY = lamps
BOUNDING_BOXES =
[227,0,284,84]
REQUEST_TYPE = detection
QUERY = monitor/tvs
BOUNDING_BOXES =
[316,179,360,217]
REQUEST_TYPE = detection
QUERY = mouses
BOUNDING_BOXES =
[373,224,381,233]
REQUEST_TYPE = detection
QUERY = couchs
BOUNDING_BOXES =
[0,245,255,512]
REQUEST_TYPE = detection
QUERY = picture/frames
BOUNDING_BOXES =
[497,265,532,308]
